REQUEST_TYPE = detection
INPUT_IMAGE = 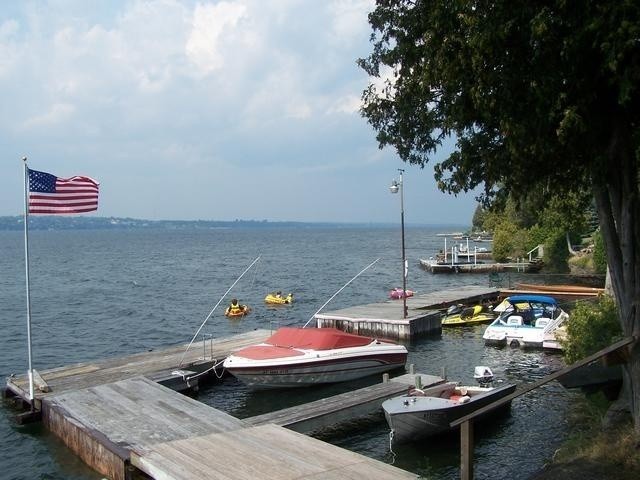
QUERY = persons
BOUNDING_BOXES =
[225,298,248,315]
[274,290,294,305]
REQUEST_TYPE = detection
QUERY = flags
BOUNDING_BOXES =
[25,165,100,215]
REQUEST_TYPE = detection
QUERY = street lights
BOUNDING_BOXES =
[388,170,409,319]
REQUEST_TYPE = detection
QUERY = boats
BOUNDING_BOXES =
[262,293,290,304]
[482,293,572,349]
[225,304,250,318]
[391,287,413,298]
[440,296,503,327]
[472,235,482,242]
[378,361,520,451]
[218,319,412,395]
